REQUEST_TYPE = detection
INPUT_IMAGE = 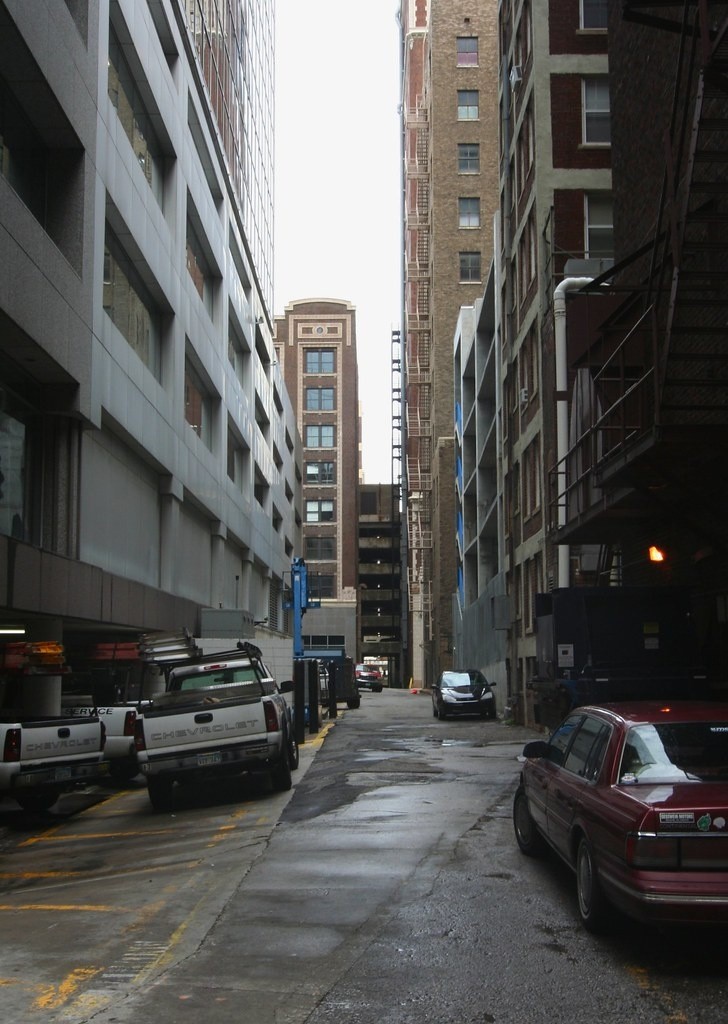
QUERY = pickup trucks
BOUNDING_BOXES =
[132,641,299,812]
[0,643,154,811]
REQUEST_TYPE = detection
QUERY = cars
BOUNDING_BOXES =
[320,659,383,709]
[430,669,497,720]
[514,701,728,934]
